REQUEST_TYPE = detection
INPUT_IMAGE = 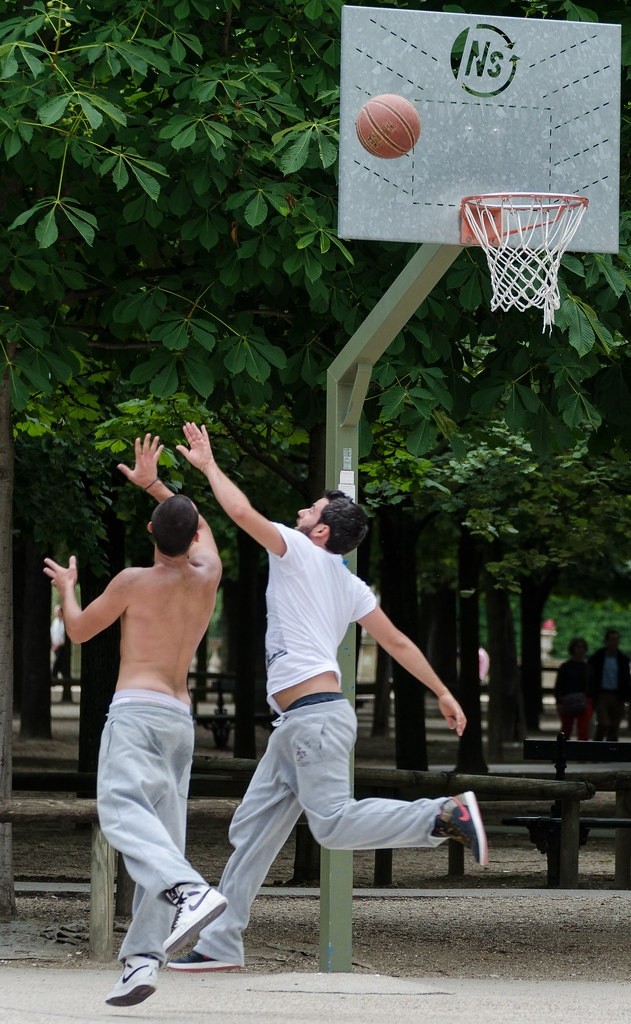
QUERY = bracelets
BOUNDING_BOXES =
[144,479,159,490]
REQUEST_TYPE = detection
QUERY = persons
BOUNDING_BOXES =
[165,422,486,972]
[478,646,488,683]
[589,630,631,742]
[50,605,65,679]
[554,637,596,740]
[42,433,229,1007]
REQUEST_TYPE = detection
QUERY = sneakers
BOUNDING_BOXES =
[433,791,488,867]
[163,883,229,955]
[104,956,160,1008]
[166,951,242,972]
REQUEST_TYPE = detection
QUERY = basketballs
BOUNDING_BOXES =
[357,95,424,160]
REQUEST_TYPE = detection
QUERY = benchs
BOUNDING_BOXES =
[188,671,280,751]
[500,732,631,886]
